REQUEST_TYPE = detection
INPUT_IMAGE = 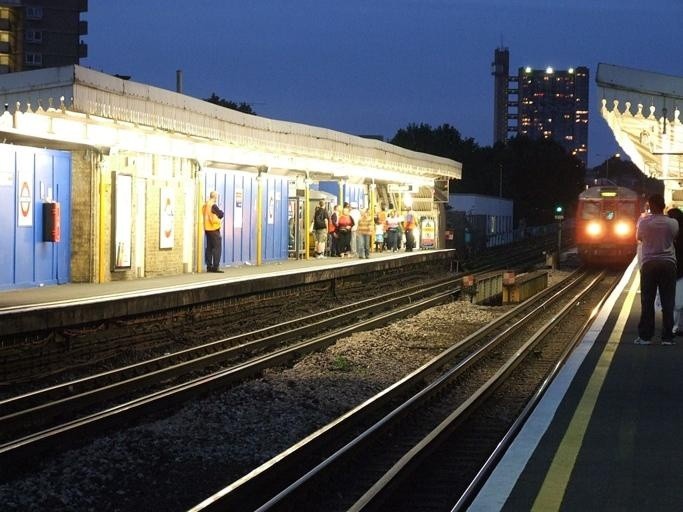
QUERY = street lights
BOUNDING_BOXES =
[594,153,608,186]
[604,150,622,186]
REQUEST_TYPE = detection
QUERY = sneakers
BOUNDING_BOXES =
[661,340,672,345]
[207,268,224,273]
[633,337,653,345]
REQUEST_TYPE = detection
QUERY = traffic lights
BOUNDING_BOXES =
[554,205,565,220]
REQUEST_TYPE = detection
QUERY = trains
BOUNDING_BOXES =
[574,184,652,268]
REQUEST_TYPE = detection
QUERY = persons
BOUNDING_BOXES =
[329,203,415,258]
[288,218,297,248]
[202,191,225,272]
[314,200,329,259]
[667,208,682,337]
[632,195,679,346]
